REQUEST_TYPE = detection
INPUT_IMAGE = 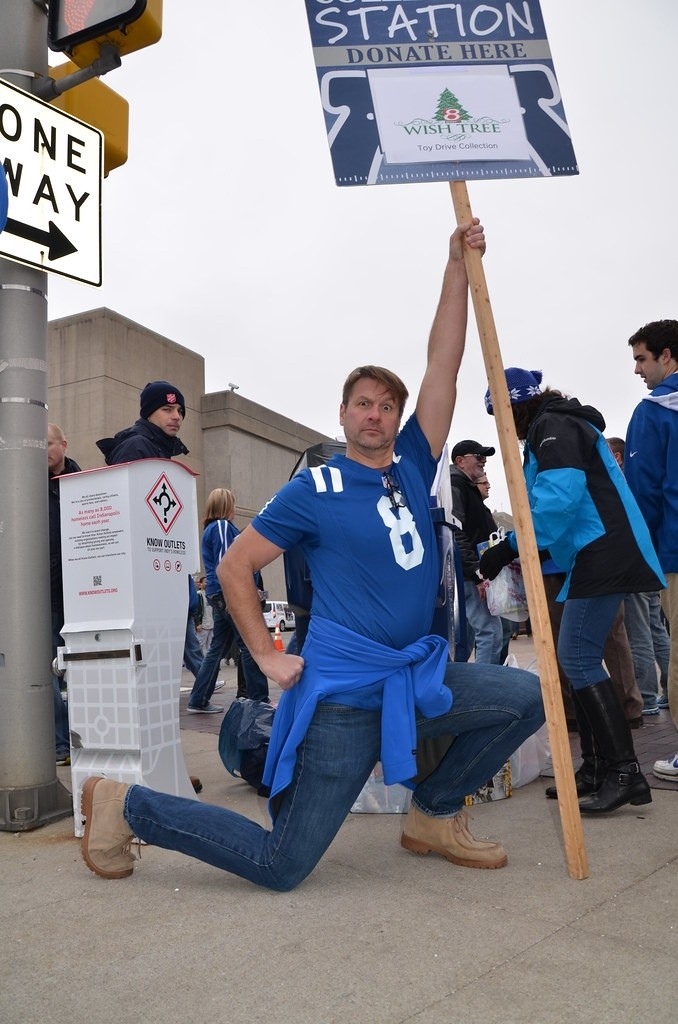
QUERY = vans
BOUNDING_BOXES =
[262,601,296,632]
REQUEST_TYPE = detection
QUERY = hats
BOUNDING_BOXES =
[451,439,495,463]
[140,381,186,419]
[484,367,542,413]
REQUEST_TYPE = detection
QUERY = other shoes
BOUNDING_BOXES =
[657,695,669,708]
[629,716,644,729]
[266,700,278,708]
[186,703,224,713]
[641,704,660,715]
[214,680,226,689]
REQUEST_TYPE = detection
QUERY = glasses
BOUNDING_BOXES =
[383,471,407,508]
[474,481,490,486]
[464,454,486,463]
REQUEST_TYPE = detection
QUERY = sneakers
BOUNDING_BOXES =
[401,802,508,868]
[653,754,678,782]
[56,749,70,766]
[80,776,142,880]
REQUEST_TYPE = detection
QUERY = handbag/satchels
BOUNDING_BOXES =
[218,696,280,797]
[476,526,528,623]
[501,653,540,788]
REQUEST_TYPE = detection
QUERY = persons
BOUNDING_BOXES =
[183,574,225,690]
[624,320,678,781]
[96,381,186,464]
[543,575,643,733]
[487,368,666,813]
[186,489,277,713]
[195,577,214,654]
[448,441,503,665]
[475,472,511,667]
[605,438,672,716]
[514,620,531,640]
[80,219,547,891]
[236,574,266,699]
[46,423,82,766]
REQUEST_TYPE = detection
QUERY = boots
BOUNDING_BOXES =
[575,679,652,814]
[545,686,597,797]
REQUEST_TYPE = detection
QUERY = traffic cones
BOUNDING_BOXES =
[273,623,288,651]
